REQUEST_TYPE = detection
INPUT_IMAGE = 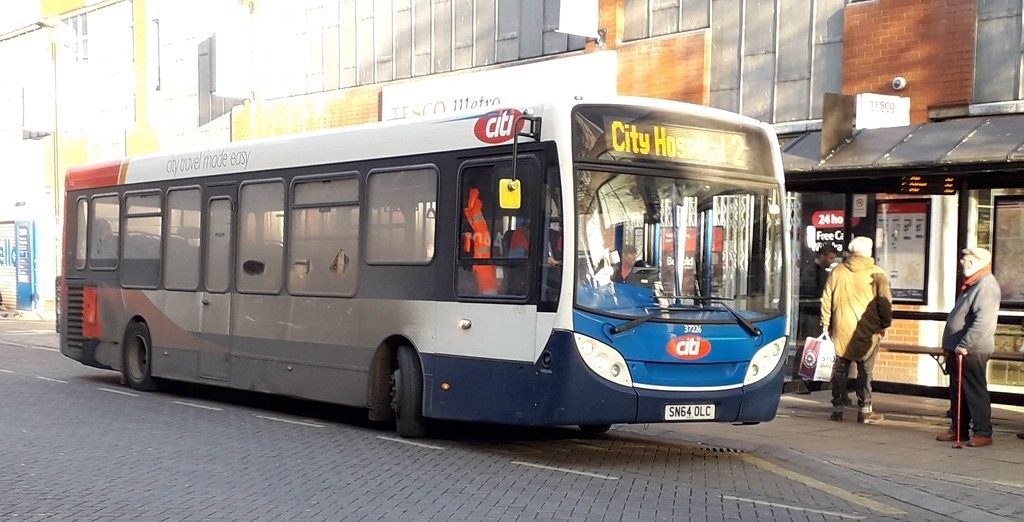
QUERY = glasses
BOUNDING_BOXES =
[959,258,978,265]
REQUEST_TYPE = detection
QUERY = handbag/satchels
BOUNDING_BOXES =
[797,332,837,382]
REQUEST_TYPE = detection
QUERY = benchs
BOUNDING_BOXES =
[798,341,1024,418]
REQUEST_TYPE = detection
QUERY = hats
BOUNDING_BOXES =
[848,236,873,257]
[962,247,991,265]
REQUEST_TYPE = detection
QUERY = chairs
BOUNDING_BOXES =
[111,230,283,290]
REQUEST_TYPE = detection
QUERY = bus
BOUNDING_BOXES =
[61,93,792,438]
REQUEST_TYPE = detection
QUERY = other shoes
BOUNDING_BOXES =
[965,434,992,447]
[830,410,843,421]
[857,414,884,424]
[935,428,963,441]
[1016,433,1024,438]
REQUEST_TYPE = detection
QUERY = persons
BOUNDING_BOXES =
[820,236,893,424]
[791,244,838,379]
[936,248,1001,447]
[507,208,563,289]
[608,245,640,284]
